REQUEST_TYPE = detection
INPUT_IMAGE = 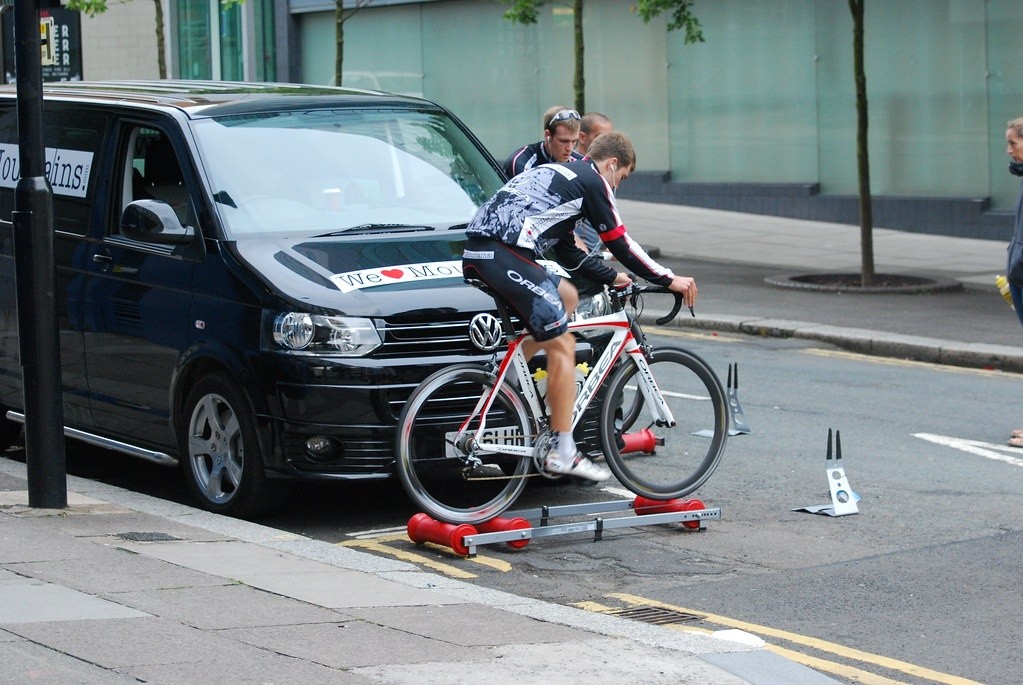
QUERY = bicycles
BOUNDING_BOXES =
[394,274,732,526]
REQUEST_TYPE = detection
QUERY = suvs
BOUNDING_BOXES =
[0,78,627,525]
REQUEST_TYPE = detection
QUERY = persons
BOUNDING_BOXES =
[462,105,698,483]
[1004,117,1023,447]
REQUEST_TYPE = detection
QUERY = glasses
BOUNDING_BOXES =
[548,109,581,128]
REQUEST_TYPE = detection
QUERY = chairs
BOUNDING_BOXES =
[141,140,192,228]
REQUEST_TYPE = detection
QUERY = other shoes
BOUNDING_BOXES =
[1008,437,1023,447]
[1011,430,1023,437]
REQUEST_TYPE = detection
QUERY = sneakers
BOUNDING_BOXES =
[544,450,610,481]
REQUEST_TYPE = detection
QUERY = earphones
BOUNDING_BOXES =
[610,164,614,171]
[547,136,551,143]
